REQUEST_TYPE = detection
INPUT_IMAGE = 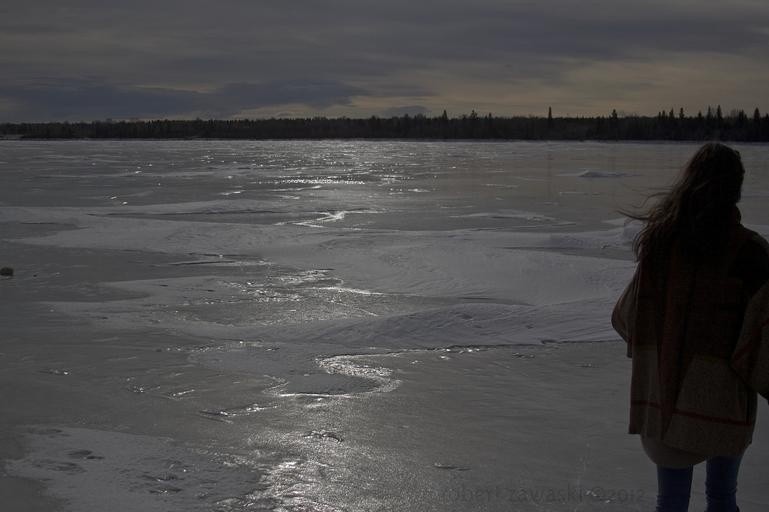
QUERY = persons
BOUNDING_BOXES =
[610,142,769,512]
